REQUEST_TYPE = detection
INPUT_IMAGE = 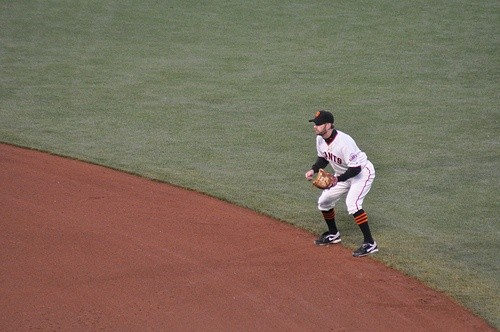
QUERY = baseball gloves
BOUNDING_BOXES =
[311,169,338,191]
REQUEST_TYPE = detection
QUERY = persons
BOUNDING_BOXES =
[305,110,378,257]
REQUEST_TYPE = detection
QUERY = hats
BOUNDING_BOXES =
[308,110,334,126]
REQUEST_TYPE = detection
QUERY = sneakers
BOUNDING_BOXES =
[313,230,342,245]
[351,240,379,257]
